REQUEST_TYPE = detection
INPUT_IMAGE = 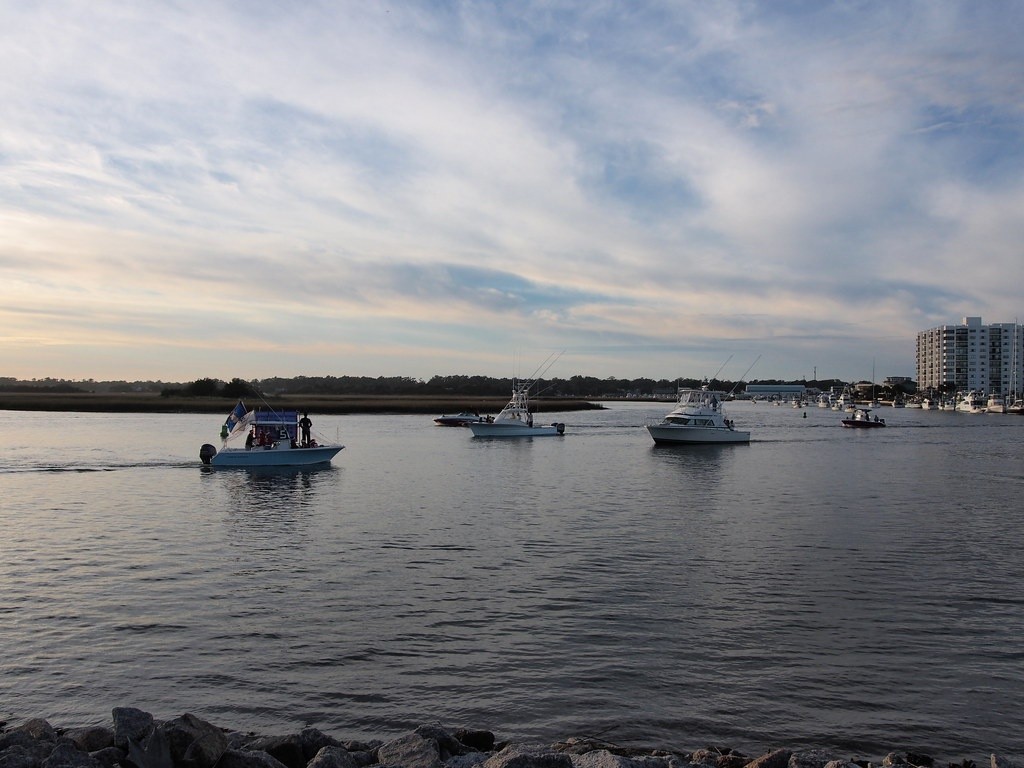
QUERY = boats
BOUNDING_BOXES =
[769,387,1024,413]
[841,409,887,428]
[433,410,494,427]
[646,351,763,445]
[466,346,569,437]
[208,385,346,467]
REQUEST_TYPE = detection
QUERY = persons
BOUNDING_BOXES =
[479,415,495,423]
[852,413,856,420]
[474,410,480,417]
[874,415,879,423]
[245,429,257,447]
[527,411,534,428]
[510,412,521,421]
[865,411,870,421]
[299,411,313,446]
[723,419,736,431]
[704,394,718,413]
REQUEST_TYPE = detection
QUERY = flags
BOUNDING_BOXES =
[226,400,256,433]
